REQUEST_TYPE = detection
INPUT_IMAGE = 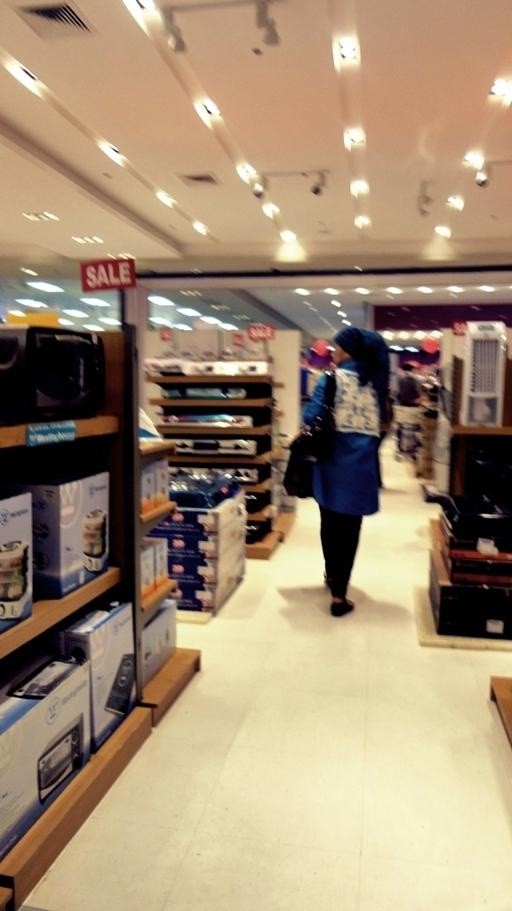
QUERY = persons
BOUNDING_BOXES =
[397,363,422,410]
[300,325,394,619]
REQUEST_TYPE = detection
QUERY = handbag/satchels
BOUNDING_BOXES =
[283,428,330,498]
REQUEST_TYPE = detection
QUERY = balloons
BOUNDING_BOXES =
[420,337,439,355]
[313,338,329,356]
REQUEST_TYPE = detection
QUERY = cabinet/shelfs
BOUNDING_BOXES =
[413,353,512,653]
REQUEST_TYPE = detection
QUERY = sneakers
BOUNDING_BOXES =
[332,599,354,616]
